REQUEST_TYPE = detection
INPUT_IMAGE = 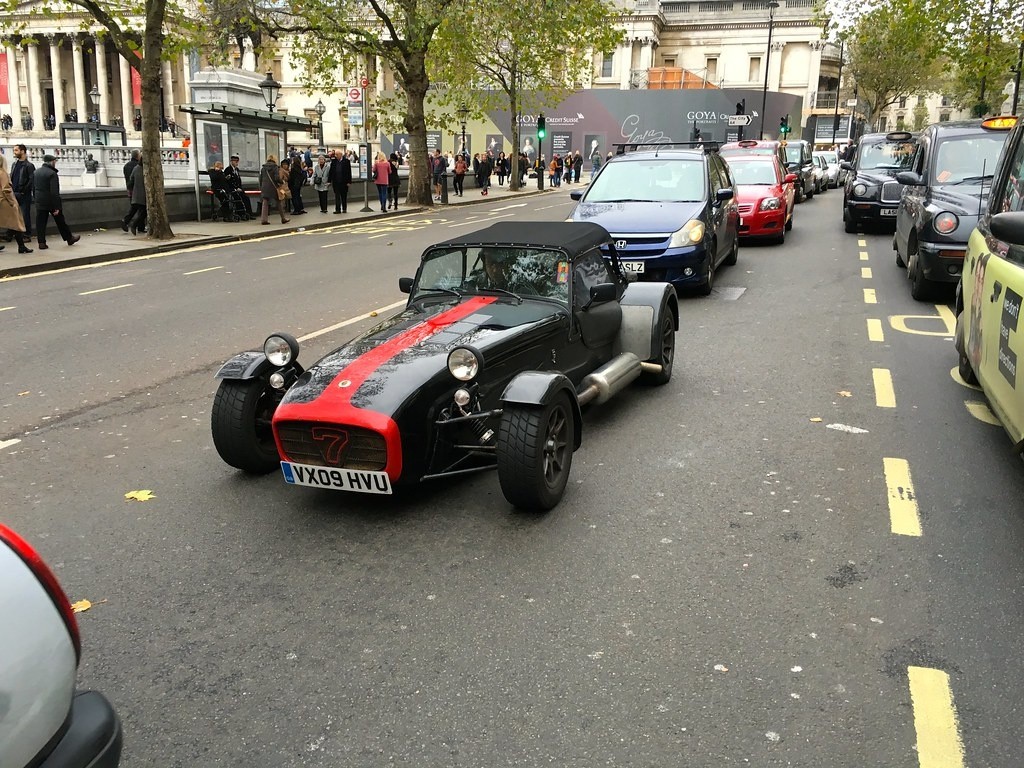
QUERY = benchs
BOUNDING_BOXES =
[206,190,262,221]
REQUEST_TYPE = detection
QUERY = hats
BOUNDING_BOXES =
[43,155,57,162]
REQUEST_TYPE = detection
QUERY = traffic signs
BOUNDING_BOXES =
[728,115,753,126]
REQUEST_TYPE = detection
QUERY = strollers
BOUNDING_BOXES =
[211,187,251,223]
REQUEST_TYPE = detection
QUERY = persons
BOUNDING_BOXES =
[473,249,541,294]
[0,153,34,253]
[522,136,537,161]
[588,140,599,159]
[474,150,495,196]
[833,140,854,162]
[497,152,530,185]
[456,135,471,156]
[32,154,82,249]
[7,144,36,242]
[326,149,353,213]
[208,161,237,222]
[224,156,256,220]
[257,153,292,225]
[343,149,360,164]
[487,137,500,157]
[287,147,313,168]
[278,158,308,214]
[534,149,614,189]
[120,147,149,235]
[3,113,169,132]
[427,149,471,201]
[372,151,404,211]
[397,138,408,157]
[310,157,332,213]
[328,150,335,158]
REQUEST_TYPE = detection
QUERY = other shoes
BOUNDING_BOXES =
[23,238,31,243]
[282,219,290,223]
[434,196,441,199]
[67,234,80,245]
[139,228,147,232]
[39,244,48,249]
[290,209,307,215]
[0,236,12,241]
[261,221,270,225]
[380,204,399,212]
[453,193,463,197]
[121,220,129,232]
[131,225,137,236]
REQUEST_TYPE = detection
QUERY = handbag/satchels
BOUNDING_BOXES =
[494,166,501,173]
[277,188,286,200]
[314,177,322,184]
[373,171,377,179]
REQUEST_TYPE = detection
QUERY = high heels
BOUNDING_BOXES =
[18,245,33,254]
[0,245,5,250]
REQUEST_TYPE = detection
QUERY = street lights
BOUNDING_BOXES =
[759,0,781,142]
[831,31,849,145]
[314,97,326,154]
[456,99,470,161]
[89,84,105,145]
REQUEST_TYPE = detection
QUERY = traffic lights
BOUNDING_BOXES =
[537,118,546,139]
[735,102,744,115]
[694,128,701,140]
[780,116,791,134]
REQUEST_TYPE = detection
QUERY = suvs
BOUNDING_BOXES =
[891,116,1021,302]
[566,139,742,297]
[840,132,921,233]
[950,106,1024,465]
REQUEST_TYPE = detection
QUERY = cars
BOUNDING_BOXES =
[209,226,681,516]
[719,136,858,203]
[718,153,799,244]
